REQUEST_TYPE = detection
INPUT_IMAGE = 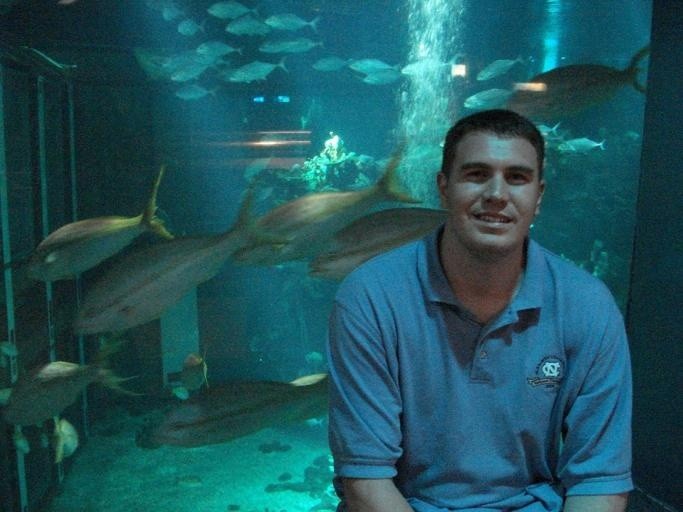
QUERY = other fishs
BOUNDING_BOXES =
[133,1,522,113]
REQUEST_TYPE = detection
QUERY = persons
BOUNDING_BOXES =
[327,108,635,511]
